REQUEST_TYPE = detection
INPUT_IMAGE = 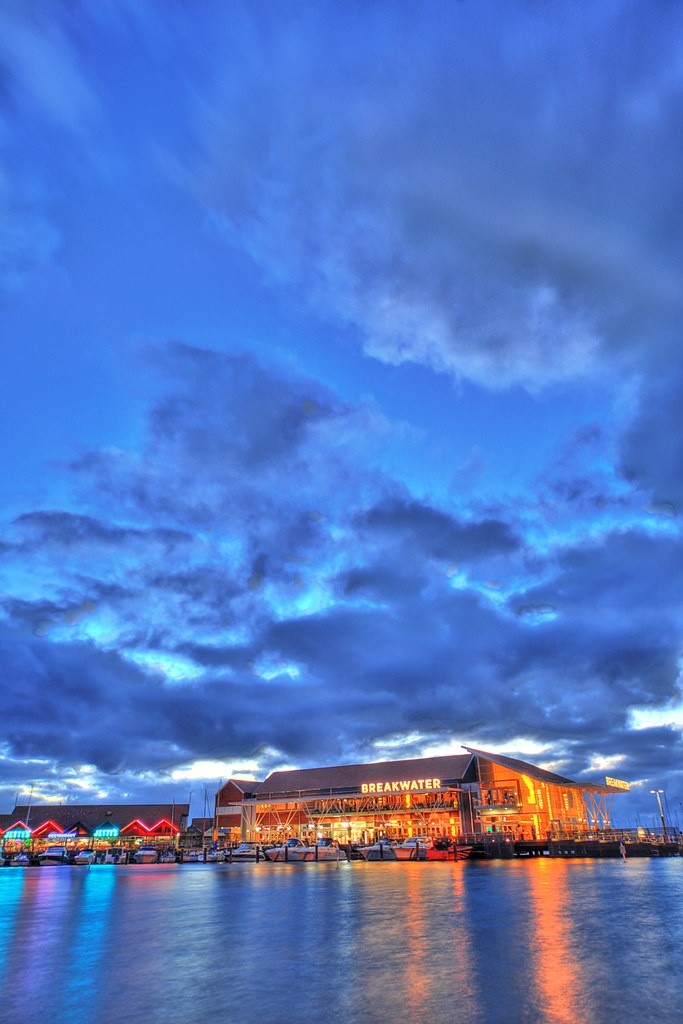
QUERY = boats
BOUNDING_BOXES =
[0,831,518,867]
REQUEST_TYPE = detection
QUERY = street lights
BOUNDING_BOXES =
[26,782,36,828]
[650,790,668,839]
